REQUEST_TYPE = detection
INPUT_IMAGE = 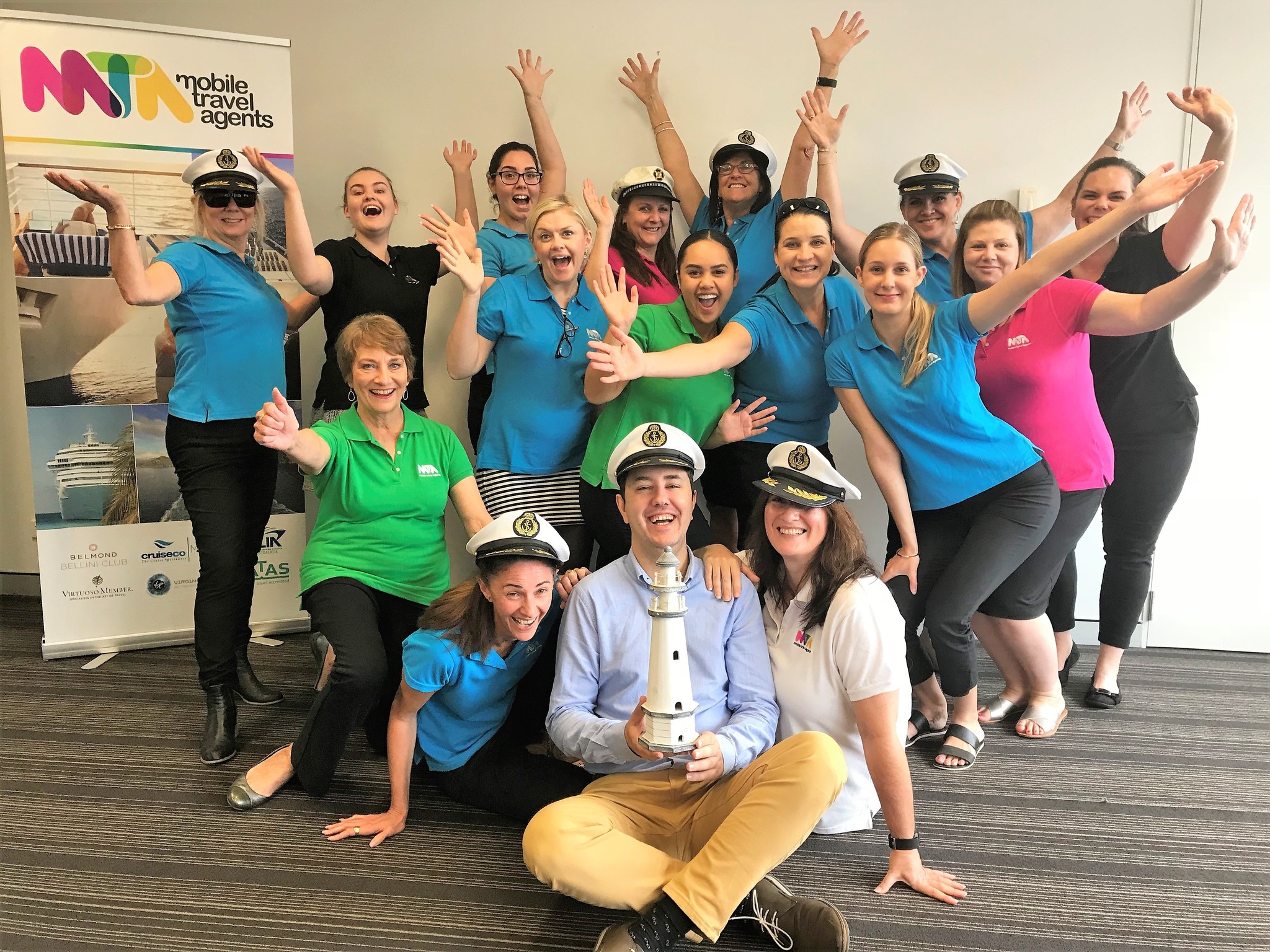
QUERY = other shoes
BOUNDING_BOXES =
[228,744,292,811]
[1084,670,1121,708]
[308,631,330,695]
[1058,640,1080,688]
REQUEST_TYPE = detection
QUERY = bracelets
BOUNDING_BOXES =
[106,223,135,231]
[816,76,837,88]
[653,120,672,132]
[896,547,919,558]
[655,127,675,137]
[818,149,838,154]
[1104,138,1124,152]
[817,158,837,165]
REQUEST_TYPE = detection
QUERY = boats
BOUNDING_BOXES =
[13,226,302,408]
[46,424,133,520]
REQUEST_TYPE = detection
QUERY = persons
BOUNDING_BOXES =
[321,507,596,847]
[521,420,851,952]
[43,150,323,766]
[824,157,1226,772]
[692,440,968,907]
[43,11,1259,812]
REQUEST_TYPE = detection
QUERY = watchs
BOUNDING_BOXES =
[888,831,921,851]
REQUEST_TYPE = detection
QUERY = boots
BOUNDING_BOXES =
[232,629,285,705]
[201,682,237,764]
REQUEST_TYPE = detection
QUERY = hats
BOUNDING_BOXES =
[893,154,968,195]
[709,130,777,178]
[612,167,681,203]
[752,442,862,508]
[181,149,263,194]
[467,510,570,568]
[607,423,706,490]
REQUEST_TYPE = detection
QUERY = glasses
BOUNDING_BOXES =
[491,172,543,185]
[716,162,760,175]
[555,317,579,358]
[200,189,256,208]
[777,197,831,221]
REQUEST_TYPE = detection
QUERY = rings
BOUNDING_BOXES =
[353,825,360,836]
[566,569,578,576]
[809,114,816,119]
[81,186,86,192]
[259,413,267,424]
[843,29,849,34]
[1139,107,1143,113]
[632,76,636,81]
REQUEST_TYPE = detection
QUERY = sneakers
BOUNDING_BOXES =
[592,918,644,952]
[728,876,850,952]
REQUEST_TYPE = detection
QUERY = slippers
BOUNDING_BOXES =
[978,695,1029,723]
[933,724,984,770]
[1016,705,1068,739]
[905,709,948,747]
